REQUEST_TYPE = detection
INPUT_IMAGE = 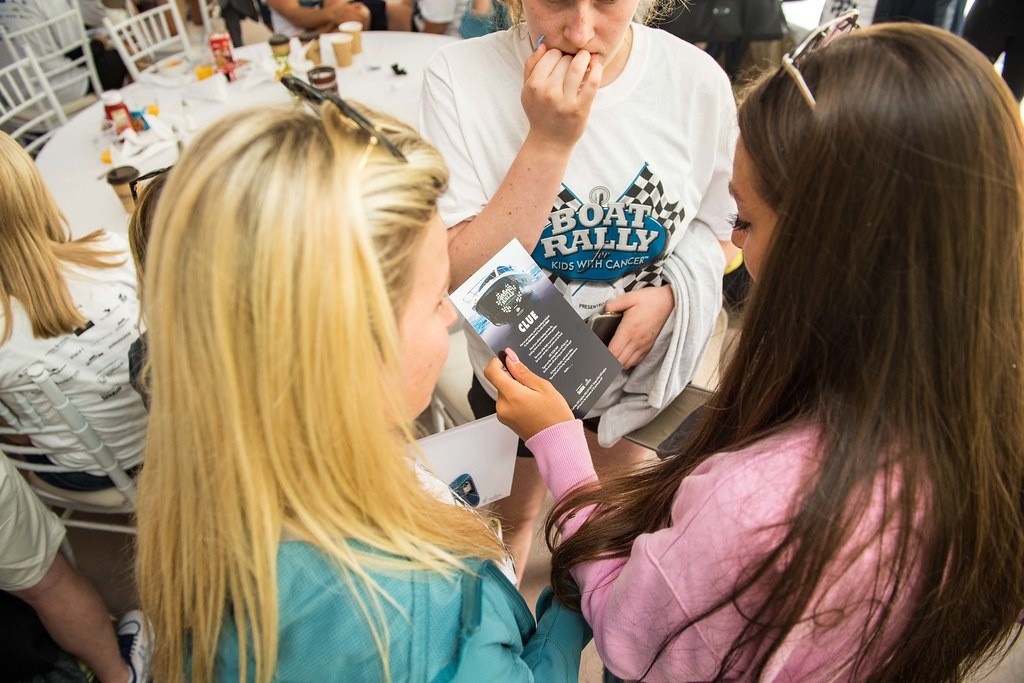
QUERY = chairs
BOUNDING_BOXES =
[0,0,224,570]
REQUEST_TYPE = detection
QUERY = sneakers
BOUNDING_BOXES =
[116,609,155,683]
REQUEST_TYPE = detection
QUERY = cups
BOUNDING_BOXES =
[307,67,338,97]
[329,33,354,68]
[299,32,322,66]
[107,166,141,213]
[338,19,362,55]
[269,35,292,59]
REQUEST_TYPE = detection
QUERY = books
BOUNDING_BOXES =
[407,414,520,508]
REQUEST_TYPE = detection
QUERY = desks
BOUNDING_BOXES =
[35,31,466,241]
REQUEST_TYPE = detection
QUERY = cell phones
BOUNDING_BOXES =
[588,311,623,348]
[450,475,480,509]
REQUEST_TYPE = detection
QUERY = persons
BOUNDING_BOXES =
[1,0,1024,117]
[421,0,740,588]
[0,133,180,682]
[137,103,594,683]
[484,23,1024,682]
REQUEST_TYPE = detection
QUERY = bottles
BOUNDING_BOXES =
[101,89,136,142]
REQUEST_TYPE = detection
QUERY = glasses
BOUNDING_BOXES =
[280,73,407,190]
[782,9,863,152]
